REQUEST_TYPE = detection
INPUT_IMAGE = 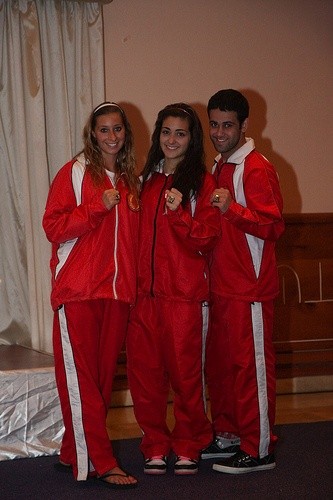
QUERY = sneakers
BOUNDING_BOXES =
[200,436,242,460]
[212,447,275,474]
[174,454,198,475]
[144,455,167,475]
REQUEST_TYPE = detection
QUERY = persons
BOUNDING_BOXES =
[43,102,142,487]
[125,102,221,474]
[201,89,284,475]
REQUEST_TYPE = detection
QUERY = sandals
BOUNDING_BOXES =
[101,466,139,487]
[59,459,74,471]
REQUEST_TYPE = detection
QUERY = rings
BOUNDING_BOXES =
[214,194,220,202]
[168,196,175,203]
[116,195,119,199]
